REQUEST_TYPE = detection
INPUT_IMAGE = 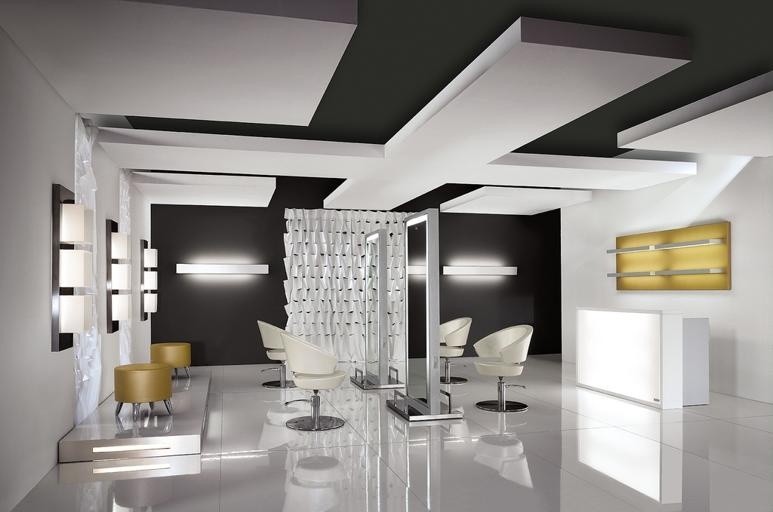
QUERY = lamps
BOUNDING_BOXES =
[49,183,269,352]
[441,266,519,276]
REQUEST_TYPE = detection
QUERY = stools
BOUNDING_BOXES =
[113,341,192,421]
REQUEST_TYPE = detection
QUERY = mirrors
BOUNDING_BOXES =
[364,207,439,414]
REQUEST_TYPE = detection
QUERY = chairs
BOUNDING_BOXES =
[439,316,534,412]
[255,318,347,432]
